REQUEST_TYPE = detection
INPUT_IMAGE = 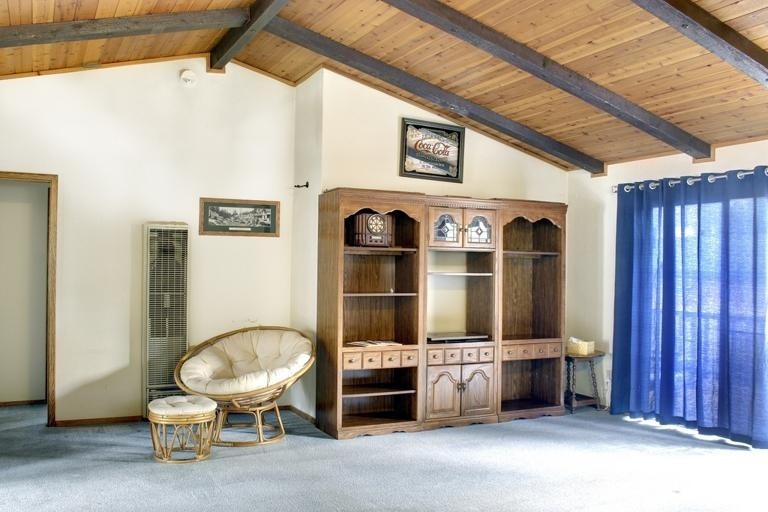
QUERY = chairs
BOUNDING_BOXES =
[174,326,316,447]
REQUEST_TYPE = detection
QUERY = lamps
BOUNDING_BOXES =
[174,326,316,447]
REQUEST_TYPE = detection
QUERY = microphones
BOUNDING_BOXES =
[147,396,217,465]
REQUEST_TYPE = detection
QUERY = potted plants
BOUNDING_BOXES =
[400,118,465,183]
[199,197,280,237]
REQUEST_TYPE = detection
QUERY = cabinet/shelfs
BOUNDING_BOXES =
[422,195,499,431]
[498,198,566,423]
[147,396,217,465]
[318,187,425,438]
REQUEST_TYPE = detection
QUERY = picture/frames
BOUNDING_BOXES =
[400,118,465,183]
[199,197,280,237]
